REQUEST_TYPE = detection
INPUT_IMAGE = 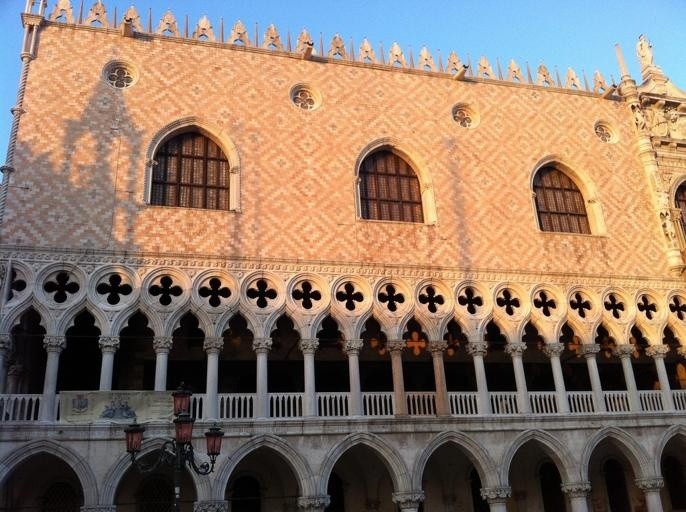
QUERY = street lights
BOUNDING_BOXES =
[122,377,225,512]
